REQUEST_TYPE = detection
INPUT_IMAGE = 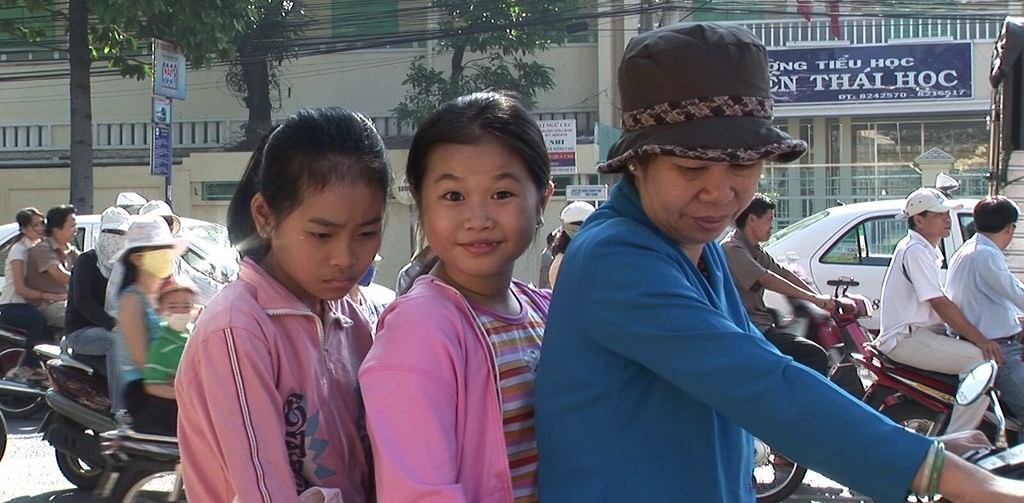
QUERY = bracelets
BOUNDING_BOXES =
[919,439,945,499]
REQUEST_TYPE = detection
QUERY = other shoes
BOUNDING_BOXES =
[18,367,48,380]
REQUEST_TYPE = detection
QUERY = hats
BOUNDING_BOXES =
[592,22,809,175]
[894,187,964,221]
[114,216,182,260]
[116,192,148,215]
[561,200,596,224]
[159,274,200,295]
[139,200,181,236]
[100,207,132,233]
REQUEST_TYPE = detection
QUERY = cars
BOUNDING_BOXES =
[1,212,398,338]
[758,172,1024,327]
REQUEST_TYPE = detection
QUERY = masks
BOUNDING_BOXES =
[161,309,193,331]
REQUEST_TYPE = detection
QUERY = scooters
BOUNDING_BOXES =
[0,302,189,503]
[747,275,1024,503]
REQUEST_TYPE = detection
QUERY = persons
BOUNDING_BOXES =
[64,207,134,425]
[549,200,596,292]
[396,220,437,298]
[26,205,82,329]
[108,215,180,425]
[115,192,181,236]
[877,186,1005,433]
[143,277,200,399]
[176,107,395,503]
[531,20,1023,503]
[538,231,557,290]
[0,207,47,382]
[356,93,556,502]
[945,196,1023,443]
[719,193,835,466]
[347,252,384,343]
